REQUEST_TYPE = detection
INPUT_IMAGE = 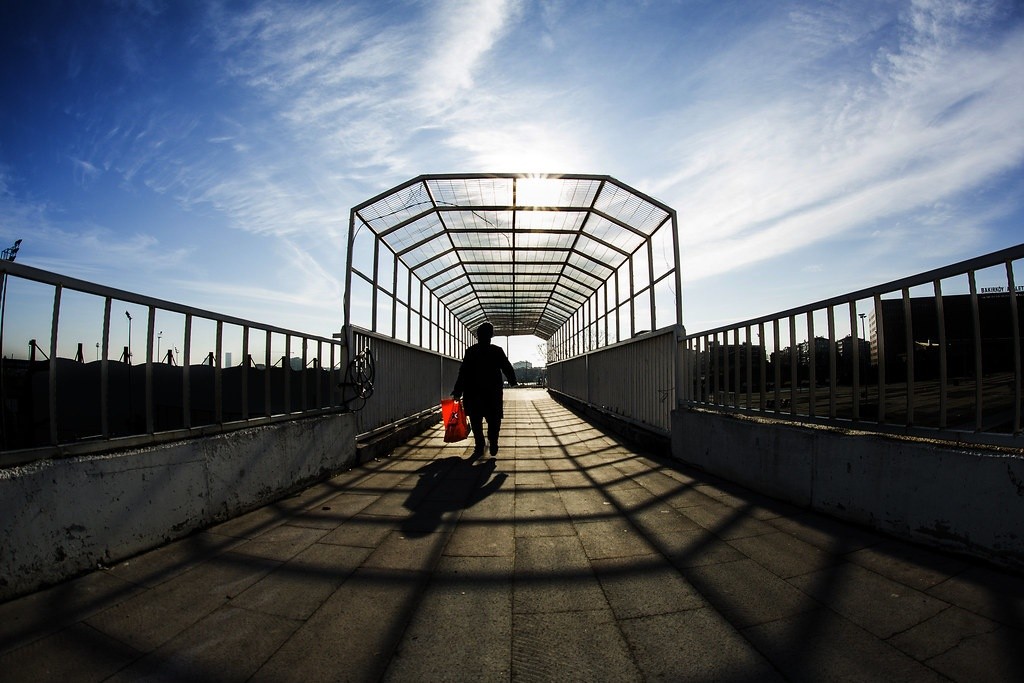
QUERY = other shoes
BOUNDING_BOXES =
[489,441,499,456]
[473,445,484,454]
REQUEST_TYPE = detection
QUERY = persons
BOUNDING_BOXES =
[450,323,519,456]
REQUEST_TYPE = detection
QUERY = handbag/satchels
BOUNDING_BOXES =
[440,397,471,443]
